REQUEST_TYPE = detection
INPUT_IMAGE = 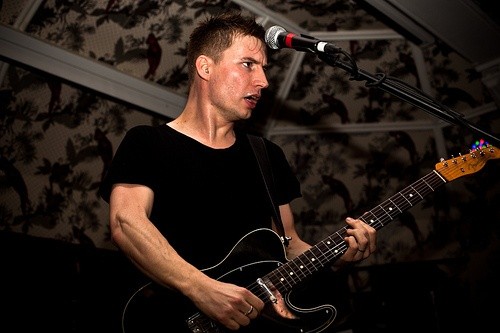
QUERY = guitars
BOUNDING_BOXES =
[120,144,498,331]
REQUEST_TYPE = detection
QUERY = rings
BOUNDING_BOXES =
[245,304,254,316]
[358,248,365,253]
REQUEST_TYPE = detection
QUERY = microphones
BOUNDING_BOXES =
[265,26,342,53]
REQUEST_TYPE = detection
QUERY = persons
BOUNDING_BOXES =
[99,14,380,330]
